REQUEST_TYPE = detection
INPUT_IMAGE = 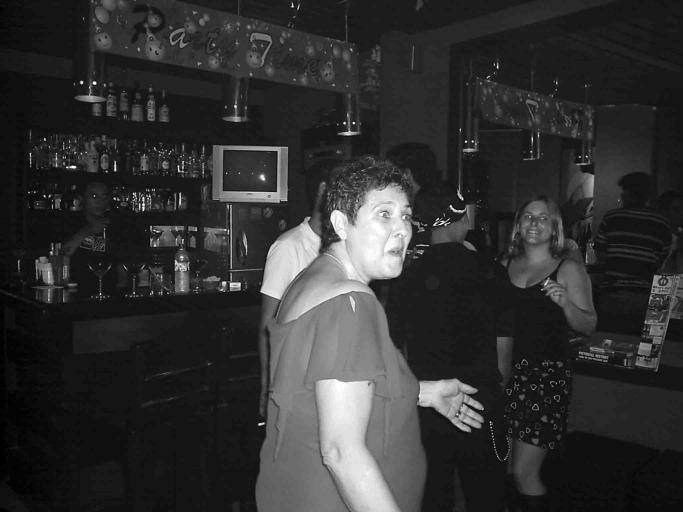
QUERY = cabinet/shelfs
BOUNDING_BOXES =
[16,113,211,225]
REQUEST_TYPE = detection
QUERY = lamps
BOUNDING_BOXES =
[73,0,107,102]
[577,78,594,166]
[222,0,250,124]
[462,59,480,154]
[338,0,361,137]
[522,66,540,160]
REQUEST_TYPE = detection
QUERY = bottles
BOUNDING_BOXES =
[147,248,166,271]
[172,241,189,294]
[28,127,209,180]
[585,237,594,265]
[76,59,170,124]
[31,240,71,285]
[190,270,202,292]
[24,177,210,213]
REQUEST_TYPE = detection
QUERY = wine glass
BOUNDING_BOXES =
[87,259,112,300]
[121,260,146,296]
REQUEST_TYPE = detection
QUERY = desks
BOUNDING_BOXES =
[569,332,683,454]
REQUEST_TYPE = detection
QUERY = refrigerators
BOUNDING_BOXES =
[192,200,293,289]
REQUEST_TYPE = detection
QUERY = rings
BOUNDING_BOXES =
[456,412,461,417]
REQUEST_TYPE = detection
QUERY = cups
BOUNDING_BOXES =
[148,272,170,296]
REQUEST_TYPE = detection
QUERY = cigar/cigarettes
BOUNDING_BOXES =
[543,276,550,288]
[257,421,266,426]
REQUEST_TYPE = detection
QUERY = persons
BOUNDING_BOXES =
[496,194,598,496]
[257,158,346,434]
[385,141,444,182]
[593,171,670,312]
[663,190,683,278]
[254,154,485,512]
[63,182,127,291]
[386,180,514,512]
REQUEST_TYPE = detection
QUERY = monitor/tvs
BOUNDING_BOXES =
[212,143,289,204]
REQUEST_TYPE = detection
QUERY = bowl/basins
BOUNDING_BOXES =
[198,279,219,293]
